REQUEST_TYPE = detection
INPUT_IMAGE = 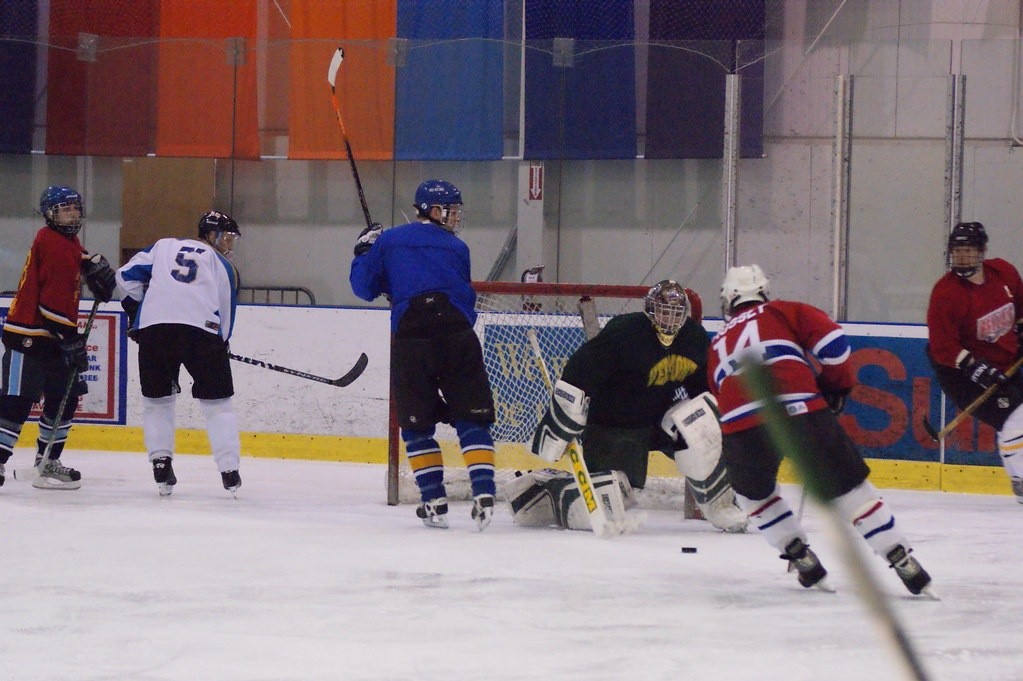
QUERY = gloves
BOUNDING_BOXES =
[125,303,140,344]
[59,335,88,372]
[960,358,1008,398]
[816,373,851,418]
[82,253,115,303]
[222,339,230,358]
[354,221,383,256]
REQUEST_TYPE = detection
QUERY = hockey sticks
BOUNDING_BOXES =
[787,484,808,572]
[14,296,100,481]
[327,46,372,227]
[921,357,1023,441]
[228,352,368,388]
[527,329,650,540]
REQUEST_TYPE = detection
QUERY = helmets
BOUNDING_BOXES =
[946,222,988,277]
[42,185,86,236]
[415,179,463,236]
[716,263,767,322]
[198,210,241,264]
[647,279,691,346]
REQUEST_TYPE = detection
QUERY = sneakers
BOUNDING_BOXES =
[417,496,450,529]
[33,454,81,490]
[222,468,242,499]
[471,493,493,531]
[780,536,835,593]
[0,464,5,490]
[887,545,941,601]
[152,455,177,497]
[1012,476,1023,504]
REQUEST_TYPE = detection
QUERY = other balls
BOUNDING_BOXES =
[682,547,697,553]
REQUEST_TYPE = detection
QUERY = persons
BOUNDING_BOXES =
[506,280,748,534]
[926,221,1023,505]
[704,264,937,599]
[117,211,242,499]
[350,180,496,528]
[0,187,118,490]
